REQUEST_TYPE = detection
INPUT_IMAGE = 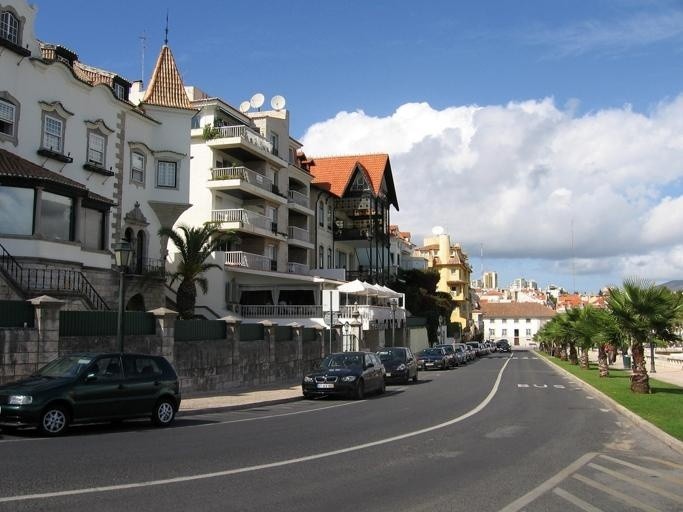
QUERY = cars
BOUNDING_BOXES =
[418,341,496,371]
[302,351,387,401]
[496,340,512,353]
[375,346,419,385]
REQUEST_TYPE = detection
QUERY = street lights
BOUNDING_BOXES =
[390,298,398,346]
[109,236,135,352]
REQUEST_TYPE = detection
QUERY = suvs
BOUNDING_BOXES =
[1,350,184,437]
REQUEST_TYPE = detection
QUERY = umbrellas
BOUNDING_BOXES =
[337,278,402,305]
